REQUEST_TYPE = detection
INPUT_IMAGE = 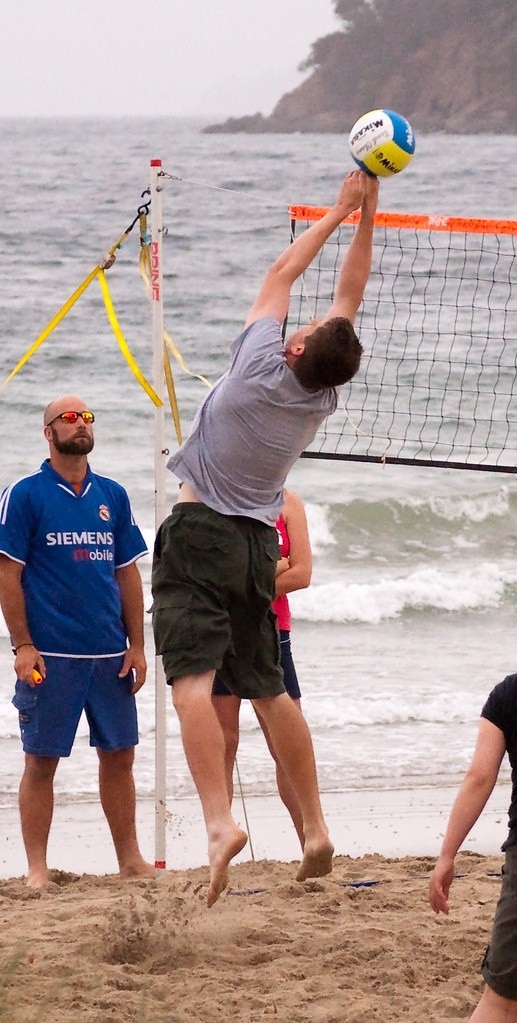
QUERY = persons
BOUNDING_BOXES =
[0,395,171,888]
[147,168,379,908]
[428,677,517,1023]
[210,487,312,853]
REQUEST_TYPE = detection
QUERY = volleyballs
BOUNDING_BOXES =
[348,107,417,179]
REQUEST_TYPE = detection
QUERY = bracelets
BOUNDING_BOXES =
[12,643,33,656]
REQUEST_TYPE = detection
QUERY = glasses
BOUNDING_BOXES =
[47,411,95,428]
